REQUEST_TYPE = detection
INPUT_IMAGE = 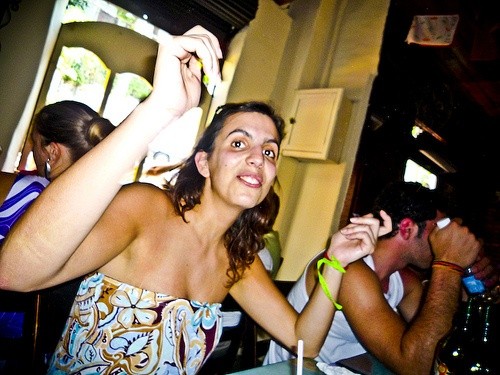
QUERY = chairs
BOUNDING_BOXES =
[0,278,298,375]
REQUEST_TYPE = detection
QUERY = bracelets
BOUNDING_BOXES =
[320,250,347,274]
[432,260,469,275]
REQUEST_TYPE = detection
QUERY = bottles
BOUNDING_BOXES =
[450,294,497,374]
[437,218,495,304]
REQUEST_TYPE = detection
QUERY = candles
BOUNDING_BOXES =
[296,340,304,375]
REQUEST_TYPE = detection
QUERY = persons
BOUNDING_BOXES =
[261,182,494,375]
[0,25,393,375]
